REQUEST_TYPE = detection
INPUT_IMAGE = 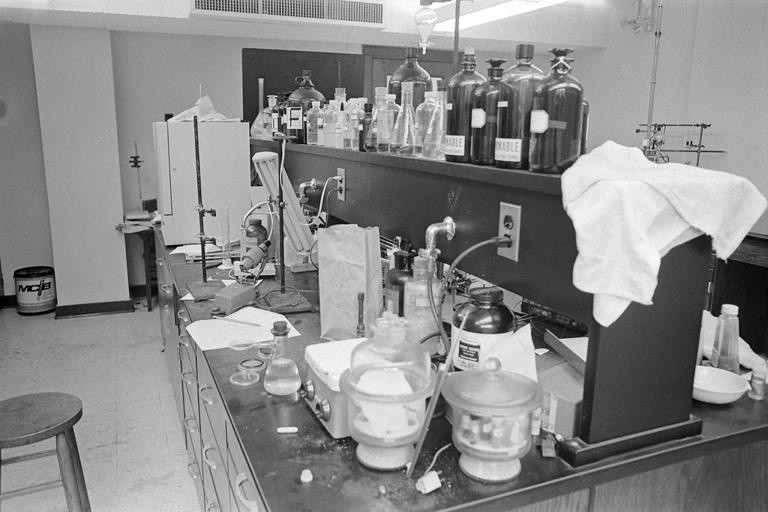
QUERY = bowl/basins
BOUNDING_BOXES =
[693,364,753,407]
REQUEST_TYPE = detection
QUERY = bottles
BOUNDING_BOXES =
[245,219,268,260]
[385,252,443,356]
[251,44,590,174]
[263,321,302,396]
[710,303,740,376]
[451,287,518,371]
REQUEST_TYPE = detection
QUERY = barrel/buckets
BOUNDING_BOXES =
[12,264,57,316]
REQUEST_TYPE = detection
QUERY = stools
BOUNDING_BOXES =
[0,392,91,511]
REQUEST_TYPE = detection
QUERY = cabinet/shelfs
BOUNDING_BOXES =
[152,223,768,511]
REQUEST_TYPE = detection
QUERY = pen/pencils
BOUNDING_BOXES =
[212,316,261,326]
